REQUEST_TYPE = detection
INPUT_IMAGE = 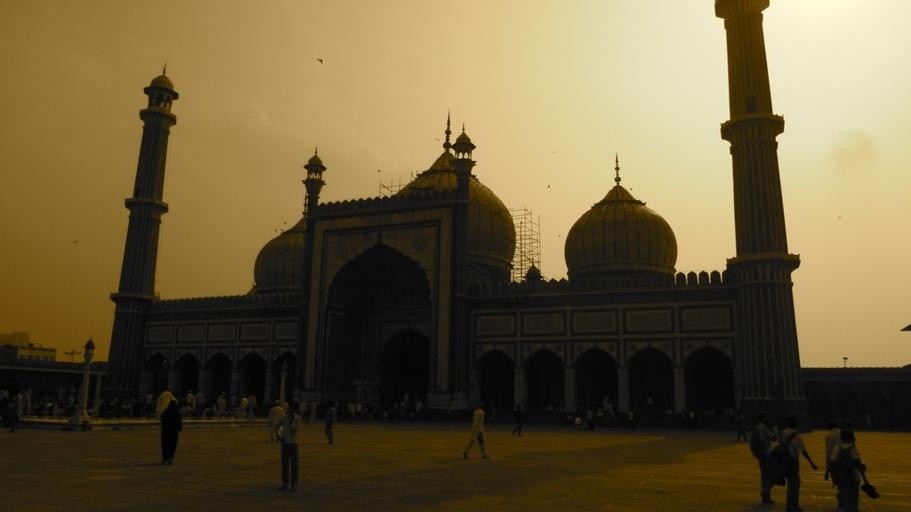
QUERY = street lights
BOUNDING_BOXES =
[842,357,847,368]
[66,335,97,425]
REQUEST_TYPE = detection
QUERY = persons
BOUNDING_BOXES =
[158,398,183,467]
[779,414,818,512]
[0,385,875,446]
[461,399,490,463]
[273,403,304,493]
[823,418,866,482]
[751,410,780,505]
[828,426,870,512]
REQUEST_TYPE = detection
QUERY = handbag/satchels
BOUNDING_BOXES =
[750,429,766,457]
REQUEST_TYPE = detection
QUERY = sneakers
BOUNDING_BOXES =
[762,496,802,512]
[278,484,298,492]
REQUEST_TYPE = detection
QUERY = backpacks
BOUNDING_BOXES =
[829,444,856,486]
[766,430,798,476]
